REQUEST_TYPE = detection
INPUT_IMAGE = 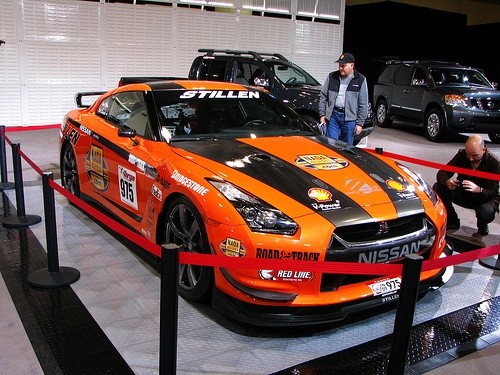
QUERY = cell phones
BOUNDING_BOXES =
[455,180,463,188]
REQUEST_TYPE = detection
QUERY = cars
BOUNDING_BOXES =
[58,80,456,327]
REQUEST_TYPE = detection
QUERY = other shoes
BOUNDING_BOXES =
[477,222,489,235]
[447,219,460,229]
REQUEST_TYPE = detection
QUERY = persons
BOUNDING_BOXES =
[319,53,369,145]
[432,135,500,236]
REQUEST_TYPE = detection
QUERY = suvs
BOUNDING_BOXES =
[373,59,500,143]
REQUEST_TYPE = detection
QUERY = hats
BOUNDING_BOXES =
[334,53,356,64]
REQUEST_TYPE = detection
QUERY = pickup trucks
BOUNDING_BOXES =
[117,48,377,149]
